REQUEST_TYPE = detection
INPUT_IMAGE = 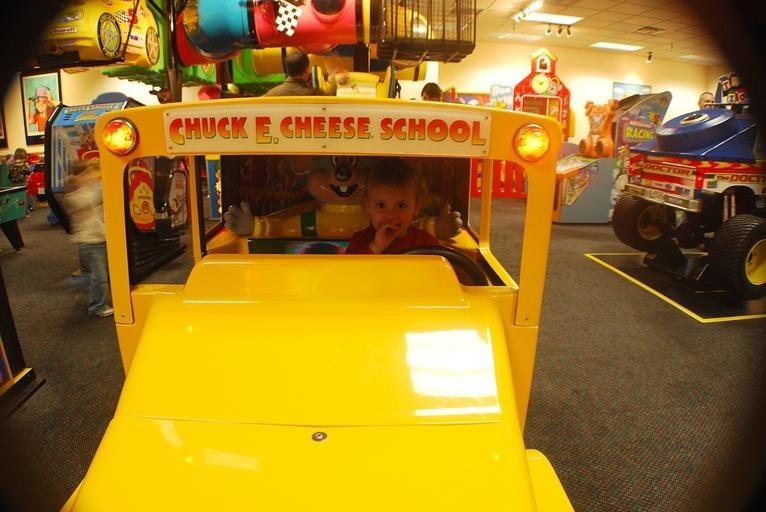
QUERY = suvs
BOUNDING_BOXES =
[613,106,766,303]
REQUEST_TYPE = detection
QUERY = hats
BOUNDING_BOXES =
[27,85,49,100]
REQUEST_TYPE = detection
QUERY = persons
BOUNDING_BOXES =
[61,160,116,319]
[258,46,341,97]
[26,85,59,135]
[8,154,32,218]
[5,148,34,212]
[698,92,716,111]
[419,81,443,101]
[344,158,463,282]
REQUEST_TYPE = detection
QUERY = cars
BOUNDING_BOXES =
[34,0,160,70]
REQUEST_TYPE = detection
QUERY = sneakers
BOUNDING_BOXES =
[87,304,113,317]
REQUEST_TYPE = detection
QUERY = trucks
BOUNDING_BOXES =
[53,89,582,509]
